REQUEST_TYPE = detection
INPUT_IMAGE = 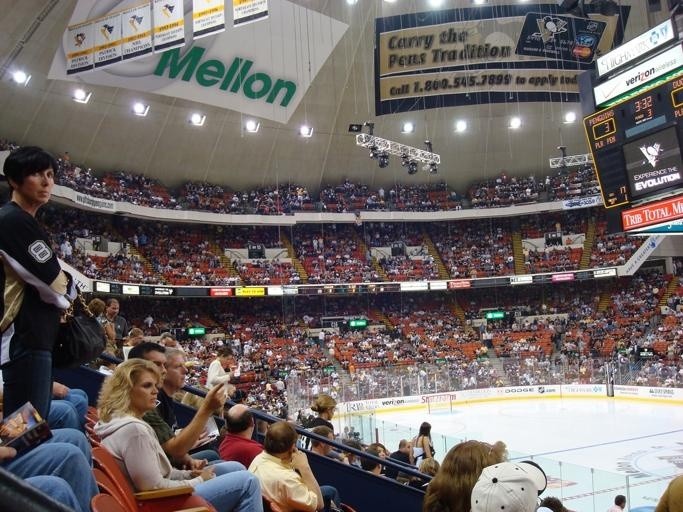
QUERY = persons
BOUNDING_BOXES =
[0,136,683,512]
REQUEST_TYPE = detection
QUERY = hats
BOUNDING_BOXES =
[471,462,547,512]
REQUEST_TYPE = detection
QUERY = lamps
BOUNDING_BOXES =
[549,145,594,178]
[349,122,441,177]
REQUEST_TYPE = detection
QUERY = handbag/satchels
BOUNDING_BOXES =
[56,309,107,366]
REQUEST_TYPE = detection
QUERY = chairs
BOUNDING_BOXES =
[82,396,350,512]
[57,159,682,395]
